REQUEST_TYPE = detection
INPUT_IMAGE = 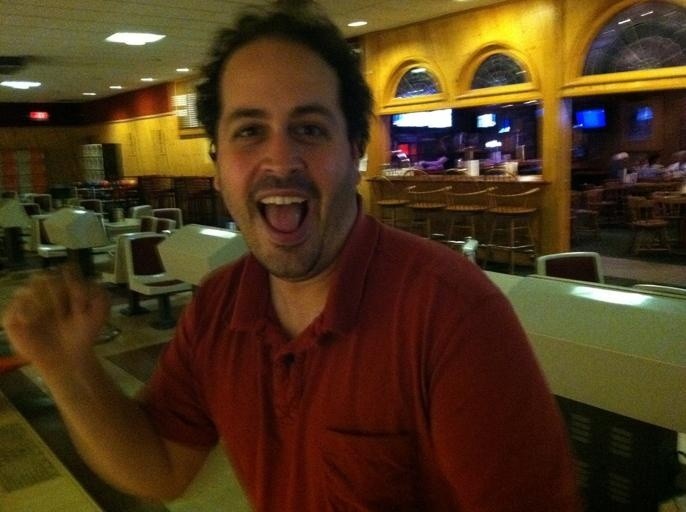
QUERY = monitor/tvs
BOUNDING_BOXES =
[574,107,608,130]
[476,112,498,130]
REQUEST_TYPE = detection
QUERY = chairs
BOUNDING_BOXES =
[1,192,195,331]
[369,169,543,275]
[572,184,686,258]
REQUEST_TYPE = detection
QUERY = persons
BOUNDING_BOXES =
[421,135,456,171]
[609,150,686,182]
[4,12,583,512]
